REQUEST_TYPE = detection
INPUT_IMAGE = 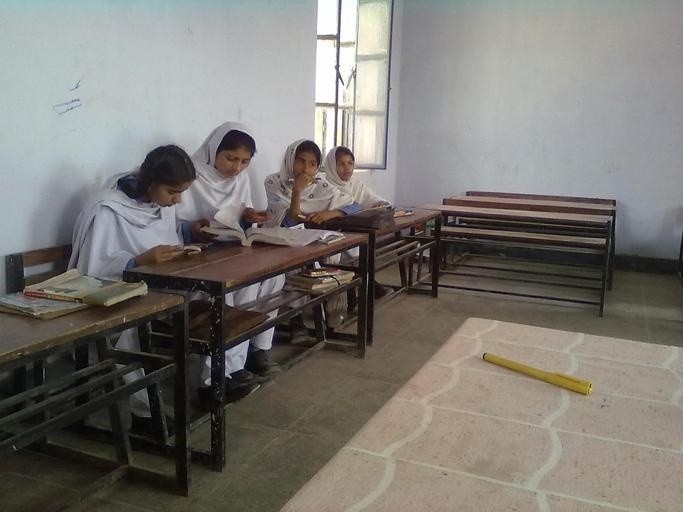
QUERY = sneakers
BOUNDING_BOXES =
[289,322,309,344]
[375,280,393,299]
[131,413,175,438]
[245,350,281,376]
[228,369,263,385]
[201,379,259,409]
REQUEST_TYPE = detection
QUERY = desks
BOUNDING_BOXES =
[299,207,442,346]
[0,275,192,512]
[74,223,368,472]
[417,204,613,317]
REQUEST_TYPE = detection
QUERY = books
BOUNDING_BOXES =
[0,268,148,320]
[199,204,345,247]
[283,267,354,296]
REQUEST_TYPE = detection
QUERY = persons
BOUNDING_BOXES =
[260,139,361,344]
[65,145,260,439]
[175,121,288,389]
[324,146,393,314]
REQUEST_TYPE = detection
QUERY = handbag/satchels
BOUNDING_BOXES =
[152,300,270,341]
[304,208,395,230]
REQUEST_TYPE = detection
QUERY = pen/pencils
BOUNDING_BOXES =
[288,178,321,182]
[483,352,592,396]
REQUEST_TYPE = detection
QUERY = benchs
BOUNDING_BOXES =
[6,245,88,436]
[458,192,616,234]
